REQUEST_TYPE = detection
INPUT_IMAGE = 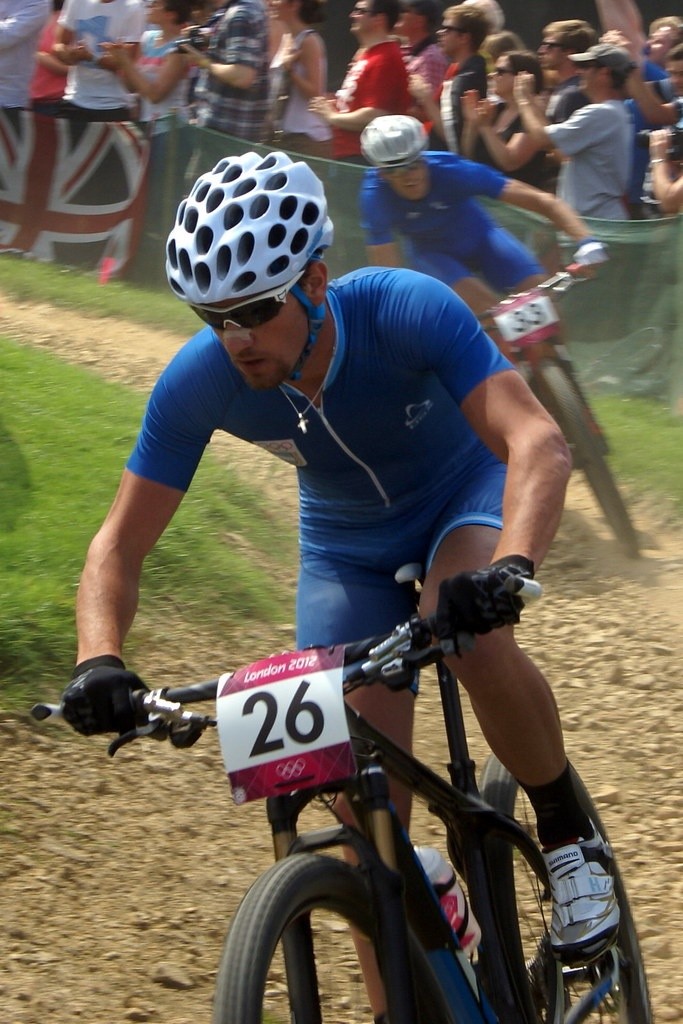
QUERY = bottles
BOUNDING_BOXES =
[409,845,483,955]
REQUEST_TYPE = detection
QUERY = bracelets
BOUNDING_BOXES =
[649,158,667,168]
[630,60,639,69]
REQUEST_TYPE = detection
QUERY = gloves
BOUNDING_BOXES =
[63,655,152,740]
[436,554,534,639]
[573,238,614,266]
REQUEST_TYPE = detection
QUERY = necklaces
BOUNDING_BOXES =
[280,374,323,433]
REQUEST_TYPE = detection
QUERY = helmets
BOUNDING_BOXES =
[166,151,334,303]
[360,115,430,167]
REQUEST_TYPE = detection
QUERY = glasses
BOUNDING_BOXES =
[376,156,420,178]
[440,26,461,33]
[354,7,371,14]
[189,267,307,330]
[496,67,511,75]
[541,41,560,48]
[577,63,595,69]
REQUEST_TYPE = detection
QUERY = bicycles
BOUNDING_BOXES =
[467,243,643,558]
[29,556,658,1024]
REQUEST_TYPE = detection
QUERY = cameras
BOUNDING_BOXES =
[637,126,683,162]
[175,29,209,53]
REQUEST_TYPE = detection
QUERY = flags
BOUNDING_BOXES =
[0,104,153,283]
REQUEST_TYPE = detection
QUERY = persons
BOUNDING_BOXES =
[307,0,545,192]
[360,114,611,368]
[0,0,334,158]
[59,149,621,1024]
[514,16,683,220]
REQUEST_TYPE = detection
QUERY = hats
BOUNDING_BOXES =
[567,43,638,70]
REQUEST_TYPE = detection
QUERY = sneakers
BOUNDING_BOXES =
[542,815,620,965]
